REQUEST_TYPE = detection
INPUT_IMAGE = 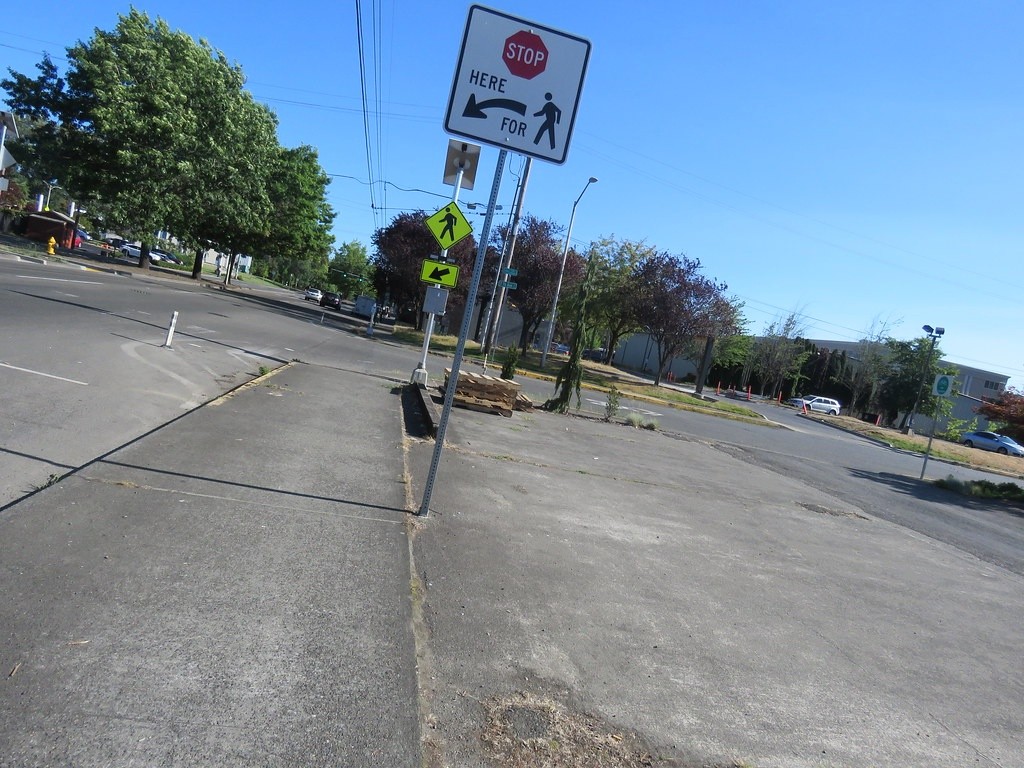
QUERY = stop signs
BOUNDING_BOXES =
[503,30,550,80]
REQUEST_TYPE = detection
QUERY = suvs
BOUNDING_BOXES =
[787,394,841,417]
[320,292,341,311]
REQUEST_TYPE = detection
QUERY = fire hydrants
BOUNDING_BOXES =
[47,235,56,256]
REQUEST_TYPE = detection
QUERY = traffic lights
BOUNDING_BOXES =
[359,273,364,283]
[343,270,348,278]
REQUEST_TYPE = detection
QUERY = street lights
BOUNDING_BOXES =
[901,324,945,434]
[539,176,599,368]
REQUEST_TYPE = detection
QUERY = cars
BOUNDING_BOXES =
[102,237,184,266]
[304,288,323,304]
[958,430,1024,459]
[74,228,93,248]
